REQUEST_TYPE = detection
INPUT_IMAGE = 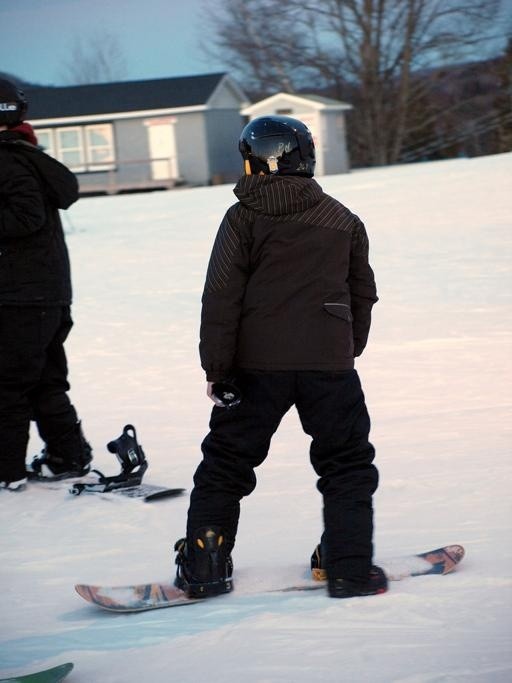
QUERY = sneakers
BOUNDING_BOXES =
[326,565,390,599]
[174,524,235,598]
[1,475,29,491]
[31,451,89,478]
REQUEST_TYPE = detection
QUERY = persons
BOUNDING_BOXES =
[1,74,98,494]
[166,110,391,599]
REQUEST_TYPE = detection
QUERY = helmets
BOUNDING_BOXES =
[236,114,317,177]
[0,77,28,120]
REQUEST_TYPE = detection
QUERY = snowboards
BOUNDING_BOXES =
[1,662,74,683]
[74,544,465,611]
[33,471,185,503]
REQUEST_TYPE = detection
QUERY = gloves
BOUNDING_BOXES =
[205,377,241,408]
